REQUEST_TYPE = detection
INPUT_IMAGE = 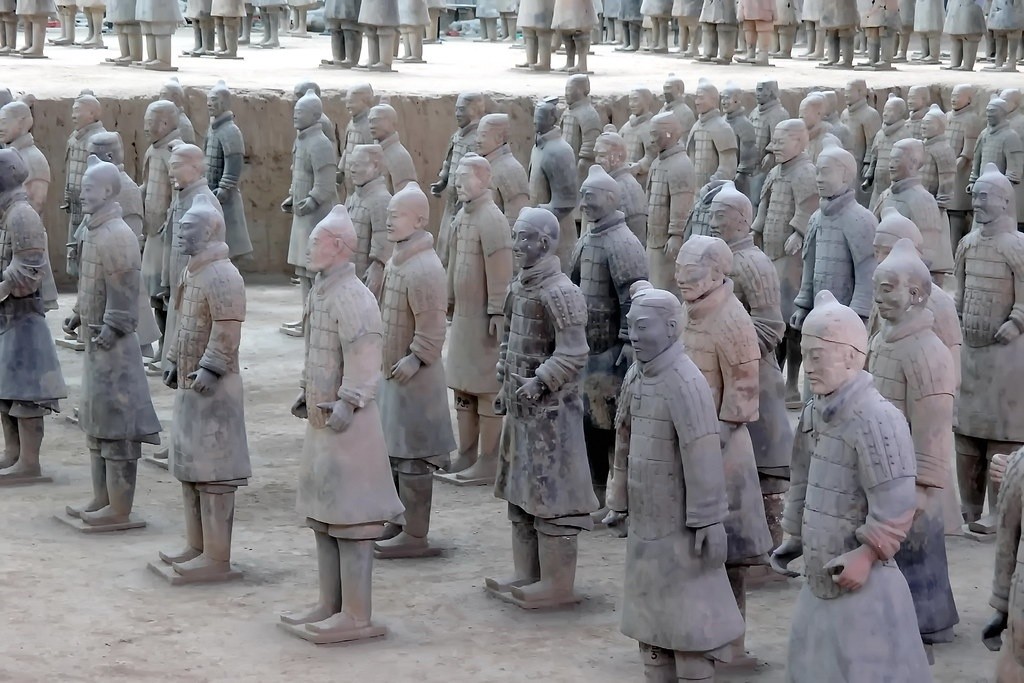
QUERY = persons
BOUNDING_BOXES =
[0,0,1024,683]
